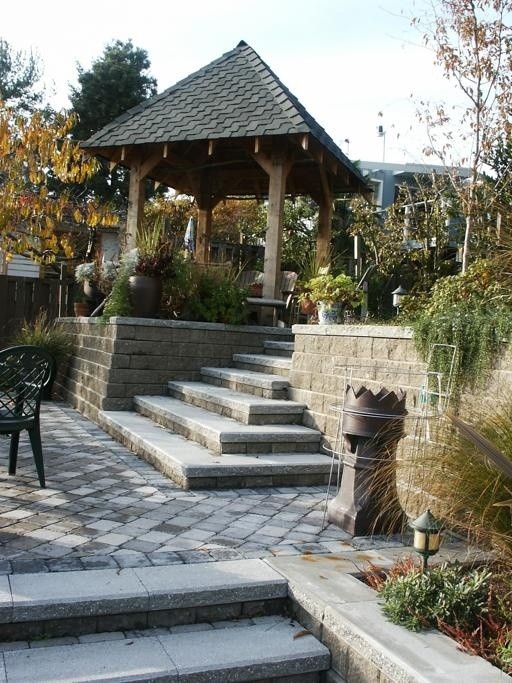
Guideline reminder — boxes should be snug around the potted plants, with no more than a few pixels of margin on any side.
[292,243,368,325]
[126,213,169,317]
[73,297,94,317]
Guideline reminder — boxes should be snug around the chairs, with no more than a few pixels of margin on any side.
[1,346,55,487]
[239,268,297,327]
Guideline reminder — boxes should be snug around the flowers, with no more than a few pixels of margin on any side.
[74,262,95,282]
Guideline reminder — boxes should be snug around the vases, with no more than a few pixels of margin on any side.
[83,280,94,296]
